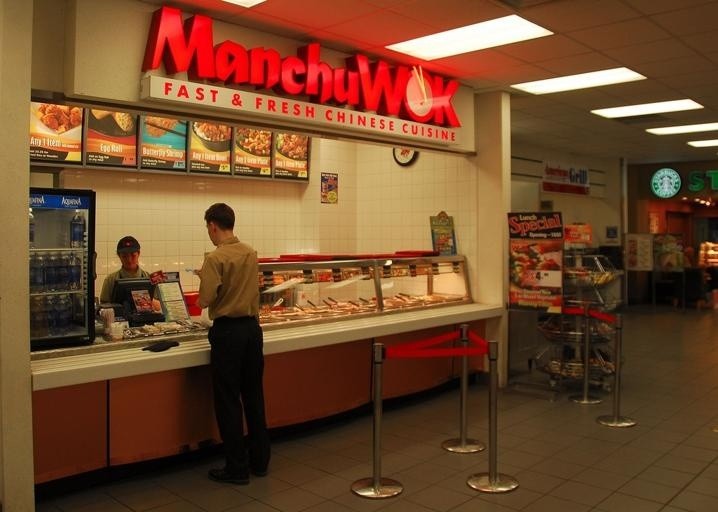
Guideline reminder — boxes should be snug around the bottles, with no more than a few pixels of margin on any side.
[29,208,35,249]
[42,253,55,291]
[28,252,43,293]
[33,294,73,337]
[68,251,80,290]
[70,209,85,248]
[56,252,67,291]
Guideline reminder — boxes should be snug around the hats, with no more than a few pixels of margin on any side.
[117,236,140,254]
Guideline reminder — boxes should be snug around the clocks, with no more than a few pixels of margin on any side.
[392,147,417,166]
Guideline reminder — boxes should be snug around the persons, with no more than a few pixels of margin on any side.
[195,203,270,485]
[99,235,161,311]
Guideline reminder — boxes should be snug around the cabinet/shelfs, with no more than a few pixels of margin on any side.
[530,253,622,392]
[27,254,504,484]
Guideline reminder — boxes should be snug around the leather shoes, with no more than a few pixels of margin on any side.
[247,463,269,477]
[208,467,249,485]
